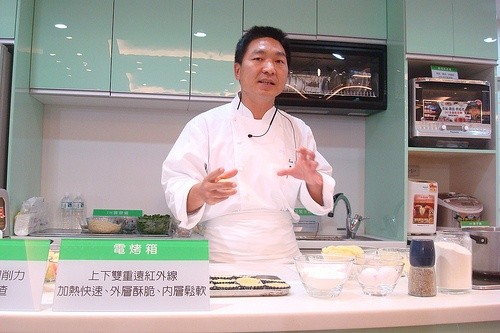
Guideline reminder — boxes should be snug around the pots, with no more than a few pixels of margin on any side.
[463,226,500,275]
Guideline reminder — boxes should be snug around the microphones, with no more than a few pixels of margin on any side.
[248,99,279,138]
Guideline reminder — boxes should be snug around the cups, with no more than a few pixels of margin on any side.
[409,238,436,297]
[435,230,473,294]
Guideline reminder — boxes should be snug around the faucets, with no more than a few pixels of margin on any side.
[327,192,370,239]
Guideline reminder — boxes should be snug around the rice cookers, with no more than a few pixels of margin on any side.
[438,192,484,231]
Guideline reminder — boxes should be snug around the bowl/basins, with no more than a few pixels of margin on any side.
[137,218,171,235]
[78,217,90,232]
[293,247,411,298]
[88,222,122,234]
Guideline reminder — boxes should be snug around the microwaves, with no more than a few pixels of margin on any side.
[274,34,387,117]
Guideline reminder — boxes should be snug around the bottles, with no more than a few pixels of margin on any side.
[61,192,85,230]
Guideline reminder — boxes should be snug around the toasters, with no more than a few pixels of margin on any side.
[409,177,438,235]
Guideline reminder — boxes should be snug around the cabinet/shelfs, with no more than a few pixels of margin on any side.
[0,0,500,235]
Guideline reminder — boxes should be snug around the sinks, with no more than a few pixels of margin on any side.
[296,234,345,242]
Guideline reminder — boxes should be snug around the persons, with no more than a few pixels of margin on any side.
[160,26,336,260]
